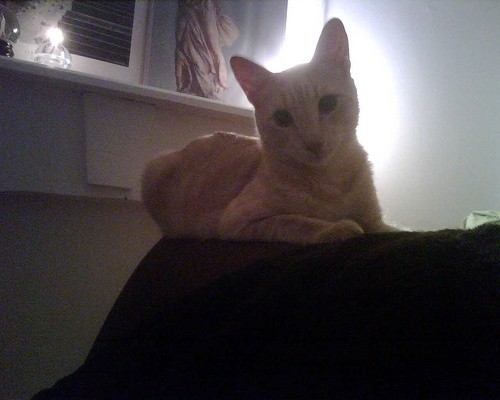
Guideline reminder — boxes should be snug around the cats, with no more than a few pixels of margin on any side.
[138,18,406,245]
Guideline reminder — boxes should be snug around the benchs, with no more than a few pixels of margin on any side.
[27,222,500,400]
